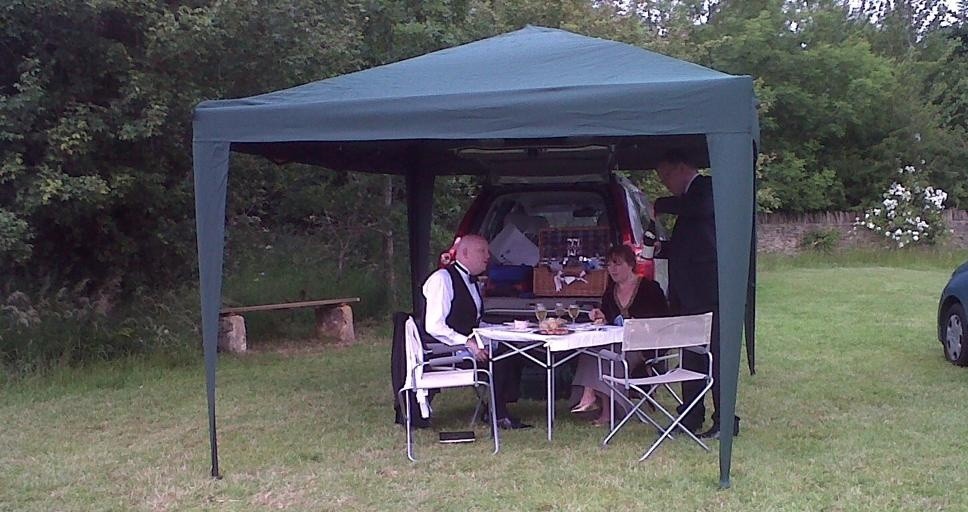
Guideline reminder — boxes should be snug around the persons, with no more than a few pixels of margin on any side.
[419,234,535,432]
[569,244,670,427]
[645,147,741,439]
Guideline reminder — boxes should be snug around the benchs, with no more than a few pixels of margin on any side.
[212,297,362,352]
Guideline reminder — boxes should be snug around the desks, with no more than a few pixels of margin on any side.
[471,323,627,444]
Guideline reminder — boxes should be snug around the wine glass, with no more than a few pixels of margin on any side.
[555,303,566,318]
[569,305,580,329]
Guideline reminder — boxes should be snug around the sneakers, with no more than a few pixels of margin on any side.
[588,419,611,428]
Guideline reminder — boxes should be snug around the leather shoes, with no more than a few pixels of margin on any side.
[694,419,739,440]
[659,417,703,436]
[481,407,533,431]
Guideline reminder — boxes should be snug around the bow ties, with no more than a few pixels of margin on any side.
[454,261,479,283]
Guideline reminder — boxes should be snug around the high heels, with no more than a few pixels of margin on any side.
[570,395,601,413]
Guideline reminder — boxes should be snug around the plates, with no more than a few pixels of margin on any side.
[508,328,533,332]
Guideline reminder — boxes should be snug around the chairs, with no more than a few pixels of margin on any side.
[397,313,501,464]
[596,311,714,463]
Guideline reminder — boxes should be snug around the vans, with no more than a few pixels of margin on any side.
[440,137,672,376]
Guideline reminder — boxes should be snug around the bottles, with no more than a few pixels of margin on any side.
[639,219,659,260]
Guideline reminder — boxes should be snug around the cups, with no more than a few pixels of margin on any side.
[535,304,548,320]
[514,319,529,329]
